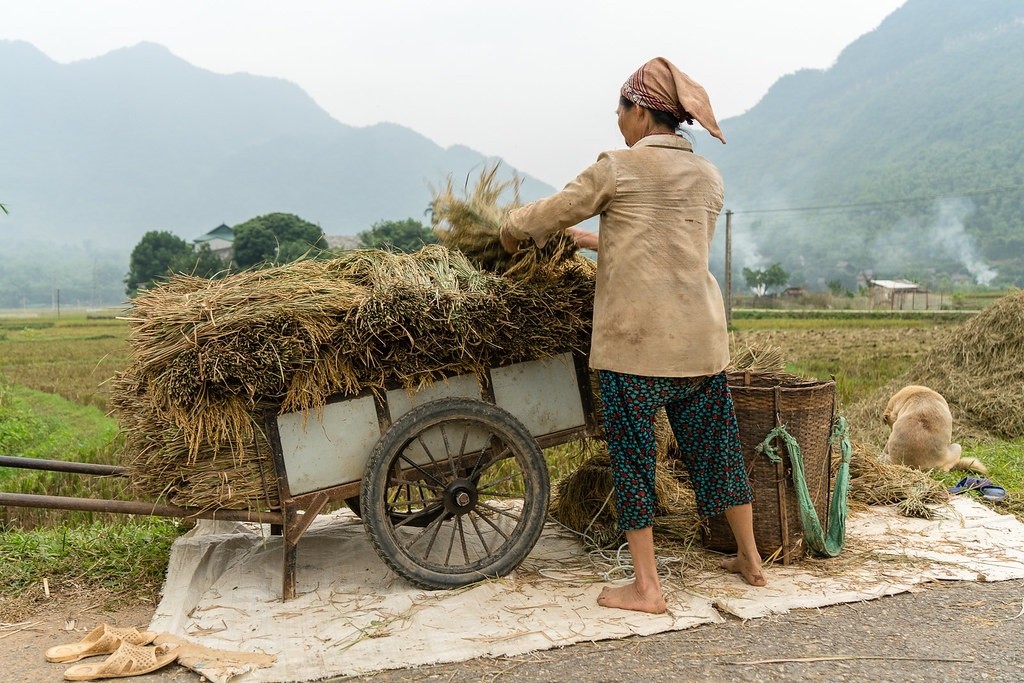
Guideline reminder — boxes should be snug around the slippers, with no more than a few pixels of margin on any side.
[980,485,1006,501]
[45,623,158,663]
[64,638,178,681]
[947,477,988,495]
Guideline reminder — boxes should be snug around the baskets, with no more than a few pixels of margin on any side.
[698,369,836,565]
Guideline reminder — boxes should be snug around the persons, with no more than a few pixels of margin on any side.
[499,57,768,614]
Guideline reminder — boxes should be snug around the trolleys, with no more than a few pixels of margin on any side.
[1,348,608,604]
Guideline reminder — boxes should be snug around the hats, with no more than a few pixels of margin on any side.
[621,57,726,144]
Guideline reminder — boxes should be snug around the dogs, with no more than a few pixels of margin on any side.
[883,385,991,476]
